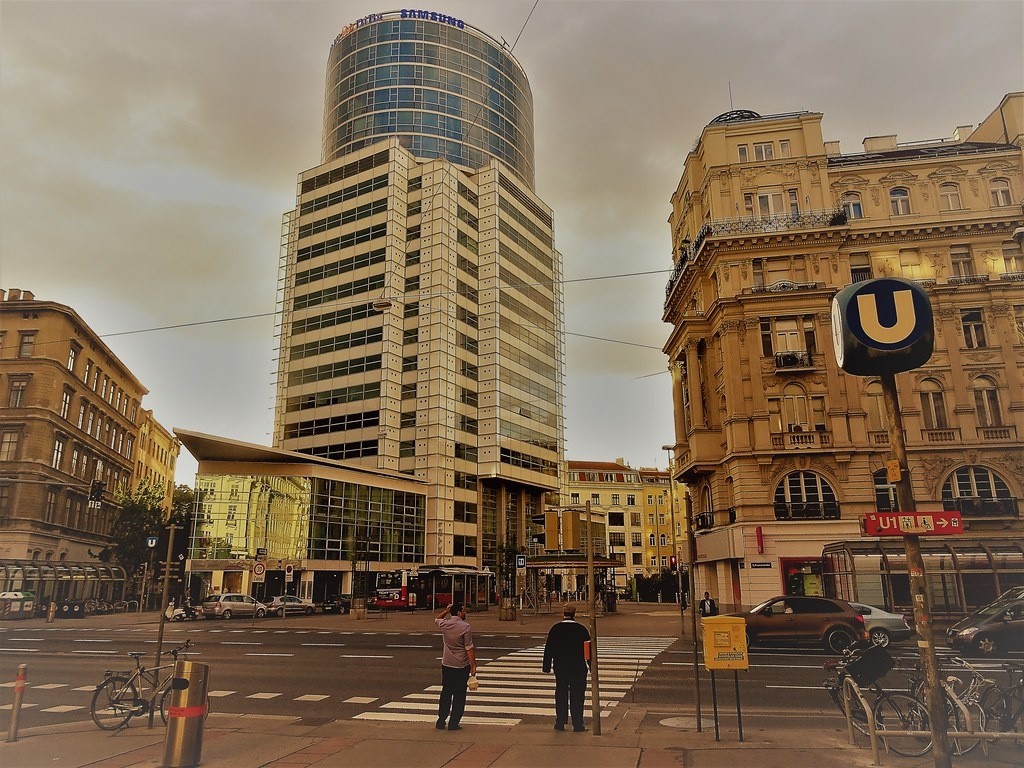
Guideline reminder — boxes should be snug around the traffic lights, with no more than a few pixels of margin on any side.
[88,480,105,503]
[669,555,676,570]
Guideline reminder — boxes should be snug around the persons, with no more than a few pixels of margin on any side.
[542,602,591,732]
[433,603,476,730]
[698,591,716,617]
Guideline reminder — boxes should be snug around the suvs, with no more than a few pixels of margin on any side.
[364,591,386,612]
[321,594,351,614]
[202,592,268,620]
[263,595,317,616]
[715,595,870,654]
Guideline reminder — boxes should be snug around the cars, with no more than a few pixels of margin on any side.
[846,601,912,648]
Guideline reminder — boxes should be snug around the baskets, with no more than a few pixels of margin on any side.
[843,643,896,689]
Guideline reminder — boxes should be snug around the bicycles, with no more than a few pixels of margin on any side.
[822,638,1024,758]
[90,637,211,731]
[74,597,115,616]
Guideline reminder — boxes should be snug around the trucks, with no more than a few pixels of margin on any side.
[997,587,1024,600]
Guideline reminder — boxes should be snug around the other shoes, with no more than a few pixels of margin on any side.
[554,723,564,731]
[436,725,445,729]
[448,725,462,730]
[574,726,585,732]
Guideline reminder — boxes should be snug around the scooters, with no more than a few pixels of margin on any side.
[165,597,200,621]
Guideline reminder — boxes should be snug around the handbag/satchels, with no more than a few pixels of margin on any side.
[467,676,479,691]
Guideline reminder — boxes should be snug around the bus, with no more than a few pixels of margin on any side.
[373,564,496,611]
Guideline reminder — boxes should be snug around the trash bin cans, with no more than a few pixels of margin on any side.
[0,592,24,620]
[20,592,36,619]
[163,660,210,767]
[46,601,56,622]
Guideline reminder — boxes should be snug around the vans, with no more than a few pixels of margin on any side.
[943,599,1024,658]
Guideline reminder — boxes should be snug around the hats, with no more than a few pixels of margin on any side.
[563,603,576,613]
